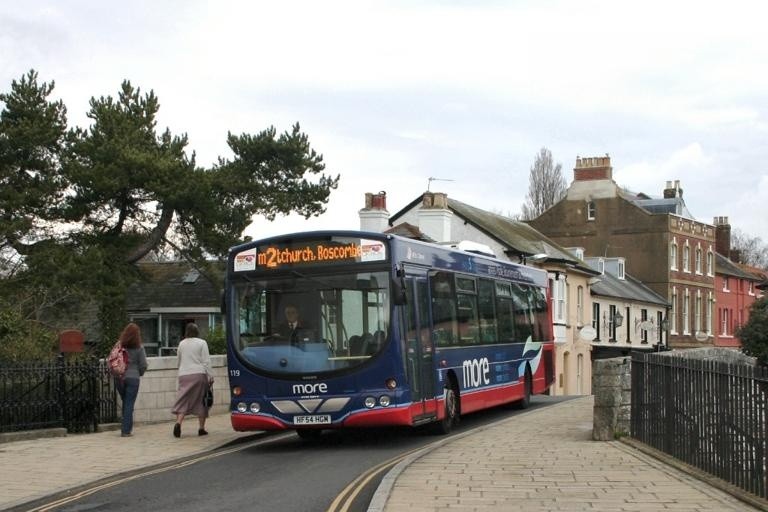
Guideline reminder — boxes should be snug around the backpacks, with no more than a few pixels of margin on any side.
[106,341,128,375]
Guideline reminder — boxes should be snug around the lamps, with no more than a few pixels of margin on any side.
[613,308,623,328]
[661,317,671,331]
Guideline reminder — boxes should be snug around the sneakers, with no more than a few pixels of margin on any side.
[121,430,134,437]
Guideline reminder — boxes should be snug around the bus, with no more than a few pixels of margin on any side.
[218,230,551,438]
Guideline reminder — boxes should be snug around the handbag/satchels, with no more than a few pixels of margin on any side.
[203,389,213,408]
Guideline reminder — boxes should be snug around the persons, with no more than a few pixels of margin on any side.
[171,323,214,437]
[110,323,148,437]
[284,304,313,332]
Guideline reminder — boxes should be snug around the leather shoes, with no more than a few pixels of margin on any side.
[173,423,181,437]
[199,429,208,435]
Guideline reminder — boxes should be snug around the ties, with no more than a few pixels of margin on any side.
[290,323,293,331]
